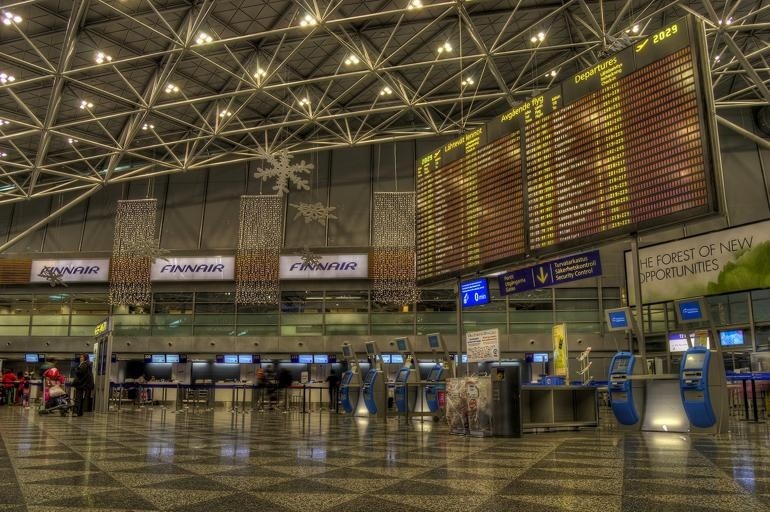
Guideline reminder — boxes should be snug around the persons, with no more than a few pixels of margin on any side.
[71,353,93,416]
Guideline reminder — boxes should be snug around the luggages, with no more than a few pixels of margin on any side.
[45,393,70,408]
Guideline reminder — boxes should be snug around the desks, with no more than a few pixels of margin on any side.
[520,384,598,432]
[406,382,445,423]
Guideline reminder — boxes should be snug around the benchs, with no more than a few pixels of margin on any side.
[108,398,135,412]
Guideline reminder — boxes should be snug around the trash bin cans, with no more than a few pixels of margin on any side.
[445,362,532,438]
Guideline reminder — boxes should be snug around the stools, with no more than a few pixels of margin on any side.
[728,385,742,416]
[597,387,612,434]
[292,394,300,412]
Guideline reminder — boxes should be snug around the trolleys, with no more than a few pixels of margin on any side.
[36,375,74,416]
